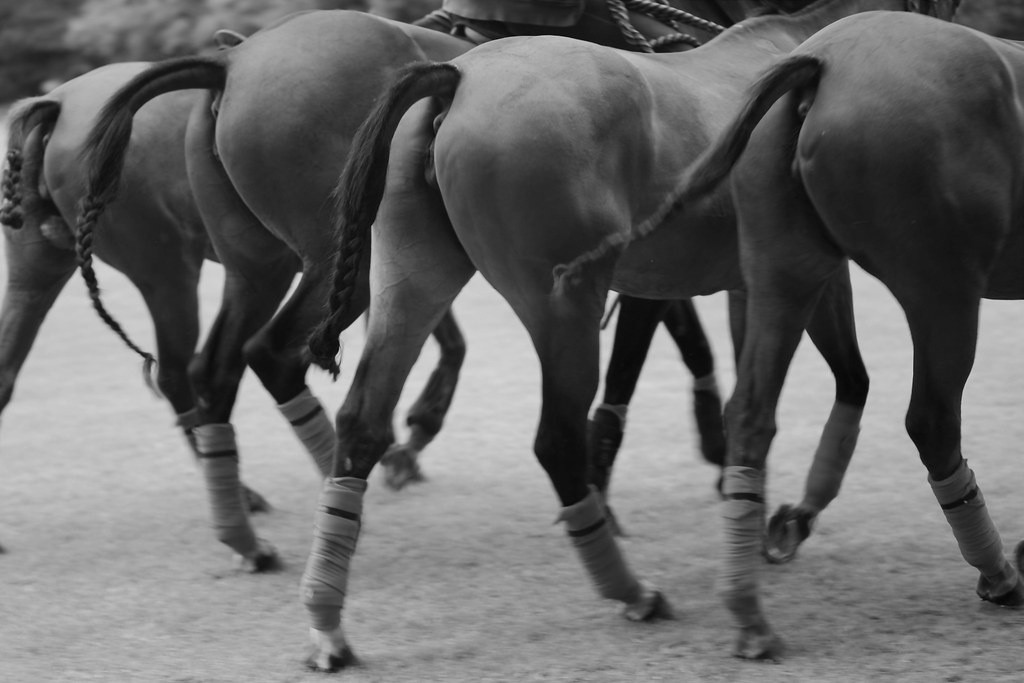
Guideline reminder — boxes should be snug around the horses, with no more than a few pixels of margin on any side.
[0,8,1023,673]
